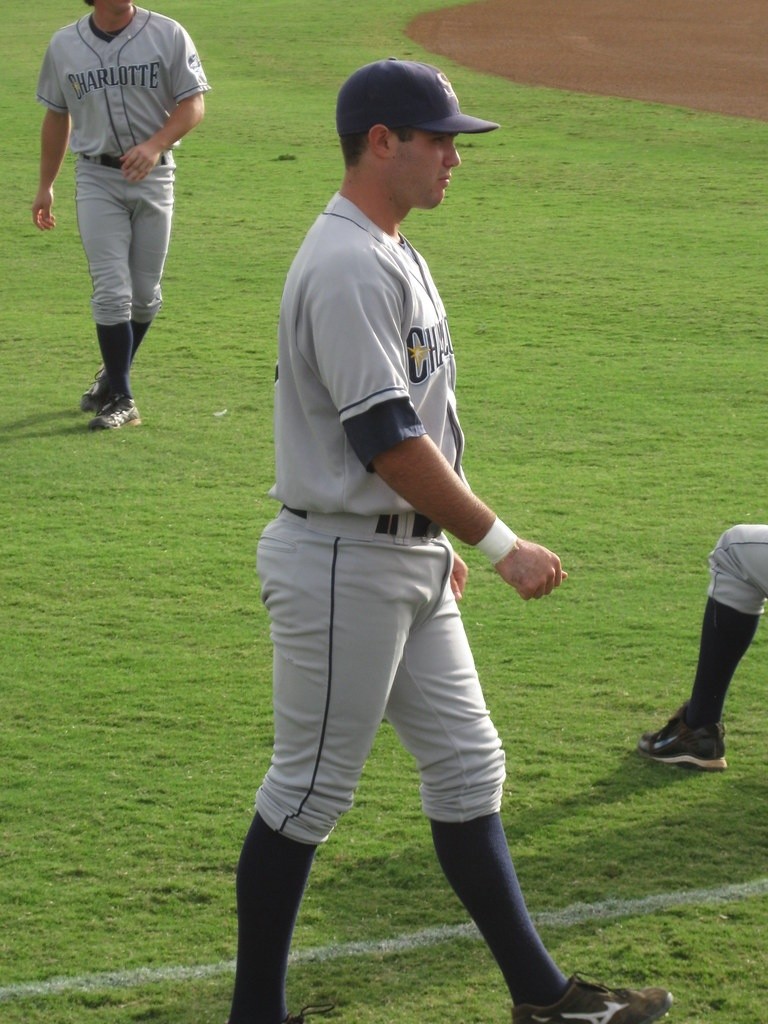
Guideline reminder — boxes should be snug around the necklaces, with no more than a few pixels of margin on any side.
[93,12,133,39]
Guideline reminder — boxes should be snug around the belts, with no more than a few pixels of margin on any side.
[82,154,165,169]
[283,503,442,539]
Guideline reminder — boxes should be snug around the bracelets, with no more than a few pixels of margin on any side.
[472,515,517,564]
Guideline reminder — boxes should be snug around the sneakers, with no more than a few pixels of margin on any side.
[511,971,673,1024]
[226,1004,334,1024]
[638,703,728,769]
[80,364,113,411]
[89,390,141,430]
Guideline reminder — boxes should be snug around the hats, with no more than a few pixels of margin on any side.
[336,57,500,136]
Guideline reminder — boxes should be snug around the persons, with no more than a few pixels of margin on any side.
[225,55,677,1024]
[637,523,767,772]
[33,0,214,434]
